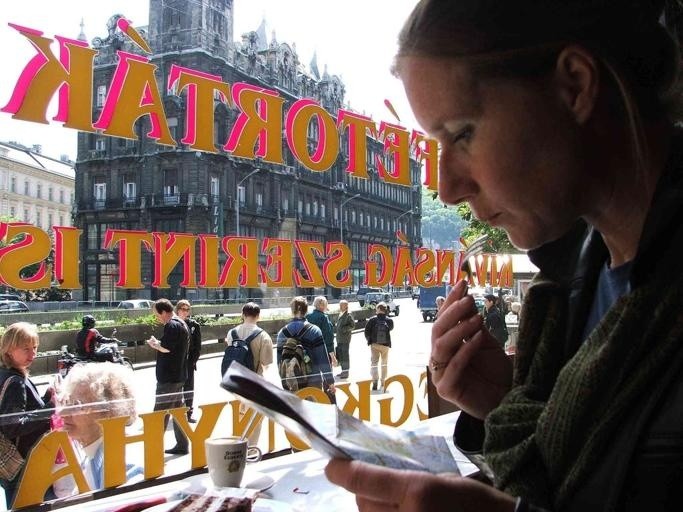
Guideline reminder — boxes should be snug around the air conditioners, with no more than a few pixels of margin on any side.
[335,181,344,190]
[286,166,294,175]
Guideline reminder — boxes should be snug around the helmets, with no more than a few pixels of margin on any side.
[82,315,95,327]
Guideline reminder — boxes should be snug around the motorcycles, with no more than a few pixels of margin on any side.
[57,327,134,378]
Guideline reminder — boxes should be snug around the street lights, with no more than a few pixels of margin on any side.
[235,169,260,300]
[395,210,412,264]
[338,194,360,298]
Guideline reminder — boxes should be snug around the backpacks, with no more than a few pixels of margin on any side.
[371,317,391,345]
[221,328,263,379]
[278,323,313,393]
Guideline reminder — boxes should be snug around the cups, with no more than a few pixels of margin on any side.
[204,437,261,489]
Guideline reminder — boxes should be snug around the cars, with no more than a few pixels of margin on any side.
[471,284,511,308]
[410,286,421,300]
[117,298,157,309]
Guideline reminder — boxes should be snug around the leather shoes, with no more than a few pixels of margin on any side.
[165,448,188,454]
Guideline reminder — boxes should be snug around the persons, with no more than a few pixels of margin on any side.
[482,293,509,351]
[324,0,681,512]
[144,298,201,454]
[276,294,355,407]
[364,301,394,392]
[1,313,145,512]
[226,302,274,455]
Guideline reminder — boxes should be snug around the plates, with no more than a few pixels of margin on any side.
[179,473,275,497]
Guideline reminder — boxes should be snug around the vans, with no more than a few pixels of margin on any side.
[0,294,23,301]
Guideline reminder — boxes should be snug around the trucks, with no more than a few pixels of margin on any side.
[418,281,454,321]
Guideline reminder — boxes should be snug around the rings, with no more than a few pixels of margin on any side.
[430,357,448,371]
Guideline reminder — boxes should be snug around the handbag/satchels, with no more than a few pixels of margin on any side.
[0,432,26,482]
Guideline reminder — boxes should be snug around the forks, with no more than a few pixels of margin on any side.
[456,232,490,302]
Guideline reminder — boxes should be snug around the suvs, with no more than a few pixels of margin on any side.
[356,287,388,306]
[364,292,399,316]
[0,299,29,312]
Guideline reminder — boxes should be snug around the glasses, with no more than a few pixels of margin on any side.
[179,307,189,312]
